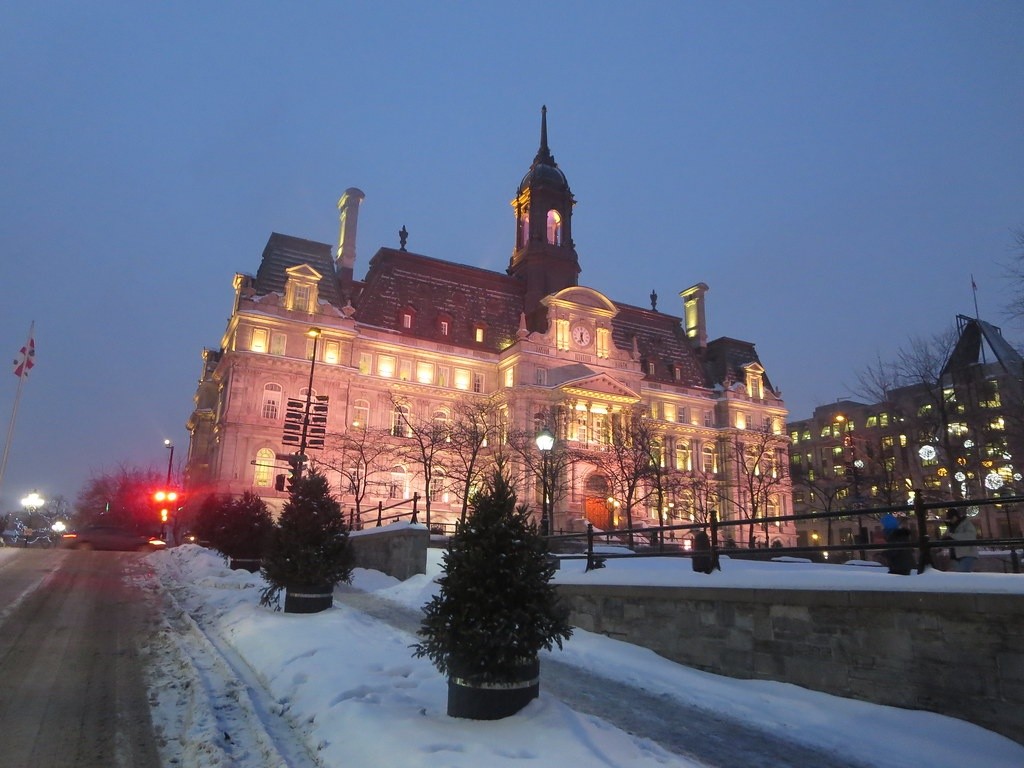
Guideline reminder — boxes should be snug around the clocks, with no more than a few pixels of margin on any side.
[572,326,590,347]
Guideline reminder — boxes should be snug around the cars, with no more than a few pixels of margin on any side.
[62,525,167,552]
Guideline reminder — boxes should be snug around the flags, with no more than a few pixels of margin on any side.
[11,329,36,378]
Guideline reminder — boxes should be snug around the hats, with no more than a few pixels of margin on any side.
[946,509,957,519]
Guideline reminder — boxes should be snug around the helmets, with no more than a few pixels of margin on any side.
[880,515,899,529]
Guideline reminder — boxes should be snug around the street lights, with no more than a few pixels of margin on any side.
[20,488,45,548]
[535,424,554,554]
[159,439,175,537]
[51,522,66,550]
[296,327,322,479]
[836,414,863,543]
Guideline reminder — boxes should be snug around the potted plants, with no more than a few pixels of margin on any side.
[260,455,354,614]
[214,491,275,573]
[406,450,577,720]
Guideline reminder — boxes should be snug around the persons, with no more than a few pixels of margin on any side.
[941,509,979,572]
